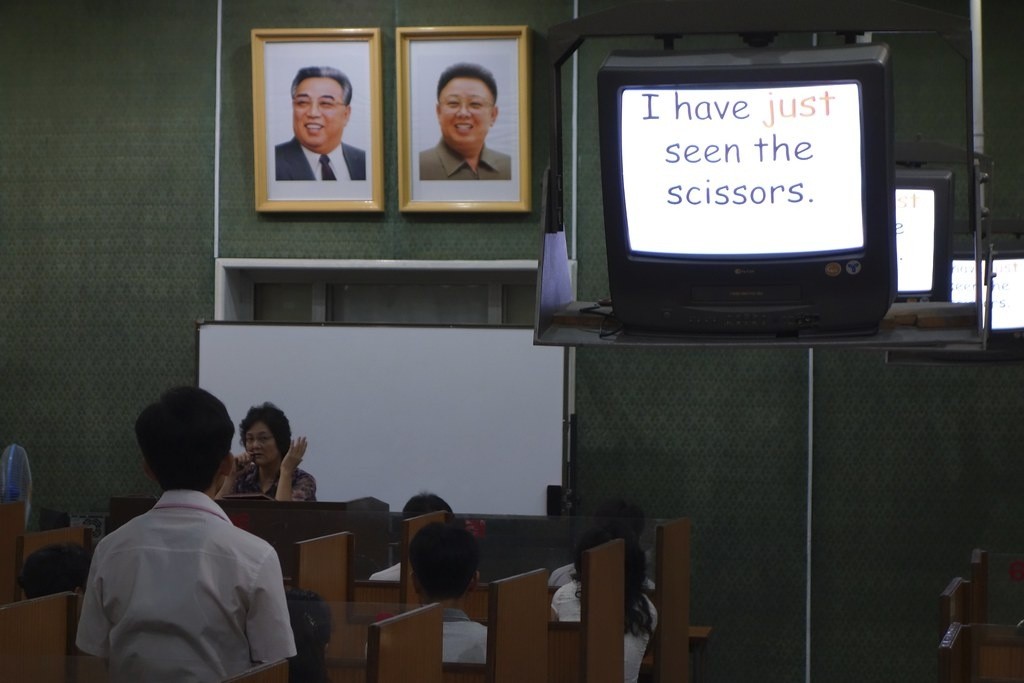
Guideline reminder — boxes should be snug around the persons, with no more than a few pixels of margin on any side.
[275,65,366,181]
[418,61,512,181]
[0,384,658,683]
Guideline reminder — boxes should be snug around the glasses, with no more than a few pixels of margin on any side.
[245,435,273,443]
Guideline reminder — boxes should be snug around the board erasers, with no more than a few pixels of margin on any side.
[546,485,562,516]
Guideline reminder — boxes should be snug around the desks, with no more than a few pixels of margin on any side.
[689,623,713,682]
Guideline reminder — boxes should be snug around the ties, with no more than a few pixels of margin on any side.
[319,153,338,182]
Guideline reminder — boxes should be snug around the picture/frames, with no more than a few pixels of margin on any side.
[395,25,532,213]
[250,27,384,213]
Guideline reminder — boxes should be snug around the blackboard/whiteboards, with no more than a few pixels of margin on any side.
[195,319,569,523]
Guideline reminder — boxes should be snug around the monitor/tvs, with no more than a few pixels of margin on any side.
[597,42,1023,340]
